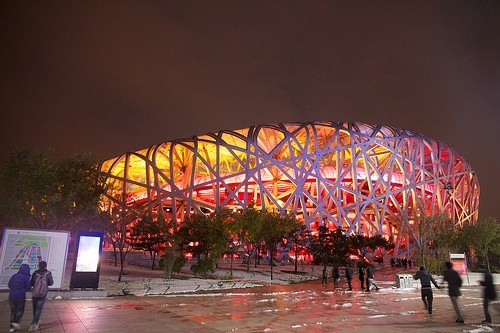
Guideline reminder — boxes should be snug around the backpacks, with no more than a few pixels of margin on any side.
[32,270,51,297]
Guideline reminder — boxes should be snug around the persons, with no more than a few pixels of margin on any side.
[477,265,494,323]
[28,260,54,331]
[442,262,464,323]
[322,254,412,292]
[412,266,440,314]
[8,264,32,332]
